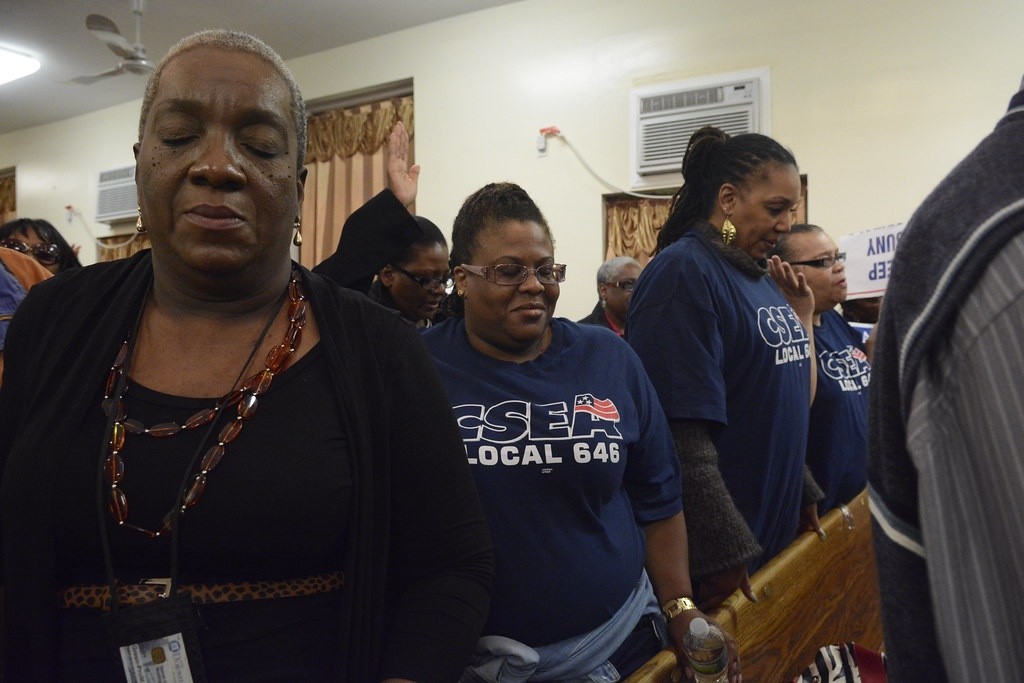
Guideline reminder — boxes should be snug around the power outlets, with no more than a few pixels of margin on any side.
[534,132,551,156]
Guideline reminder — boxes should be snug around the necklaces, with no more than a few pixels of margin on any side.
[103,267,307,537]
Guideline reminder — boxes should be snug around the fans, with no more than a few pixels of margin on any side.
[69,0,158,85]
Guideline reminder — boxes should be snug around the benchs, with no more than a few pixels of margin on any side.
[621,486,886,683]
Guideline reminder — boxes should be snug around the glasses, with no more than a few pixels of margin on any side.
[395,265,454,291]
[461,263,567,285]
[789,253,846,268]
[0,239,62,265]
[604,280,635,292]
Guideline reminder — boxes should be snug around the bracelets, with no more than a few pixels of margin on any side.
[661,597,698,623]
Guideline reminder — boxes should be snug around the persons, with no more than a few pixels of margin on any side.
[869,76,1024,683]
[625,124,827,612]
[423,182,744,683]
[760,222,880,517]
[575,256,643,342]
[0,30,497,683]
[0,218,85,380]
[310,121,452,334]
